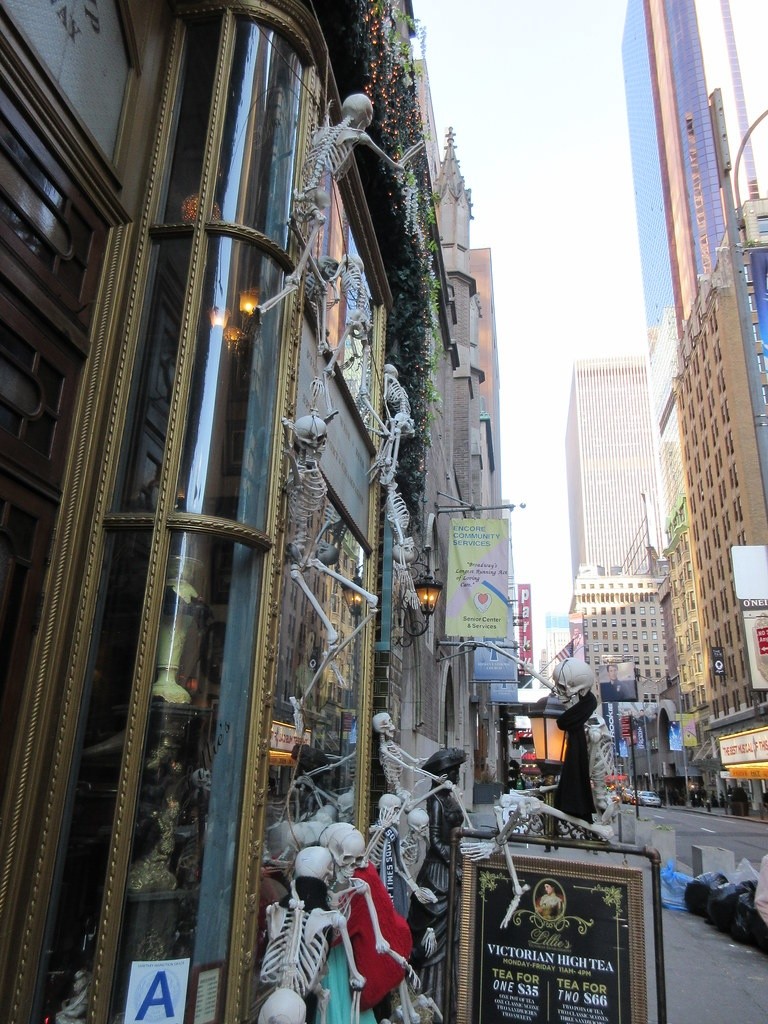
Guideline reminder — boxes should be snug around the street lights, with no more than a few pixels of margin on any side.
[676,676,697,809]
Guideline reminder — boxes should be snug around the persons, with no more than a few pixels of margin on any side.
[639,710,650,726]
[600,665,636,701]
[539,880,562,914]
[657,785,736,810]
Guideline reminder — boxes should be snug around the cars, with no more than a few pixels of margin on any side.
[620,788,661,807]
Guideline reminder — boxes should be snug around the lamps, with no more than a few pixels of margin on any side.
[391,569,443,649]
[210,285,261,358]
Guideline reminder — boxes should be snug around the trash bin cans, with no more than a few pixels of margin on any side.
[734,802,749,816]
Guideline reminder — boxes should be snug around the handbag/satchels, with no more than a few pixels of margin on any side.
[695,794,697,798]
